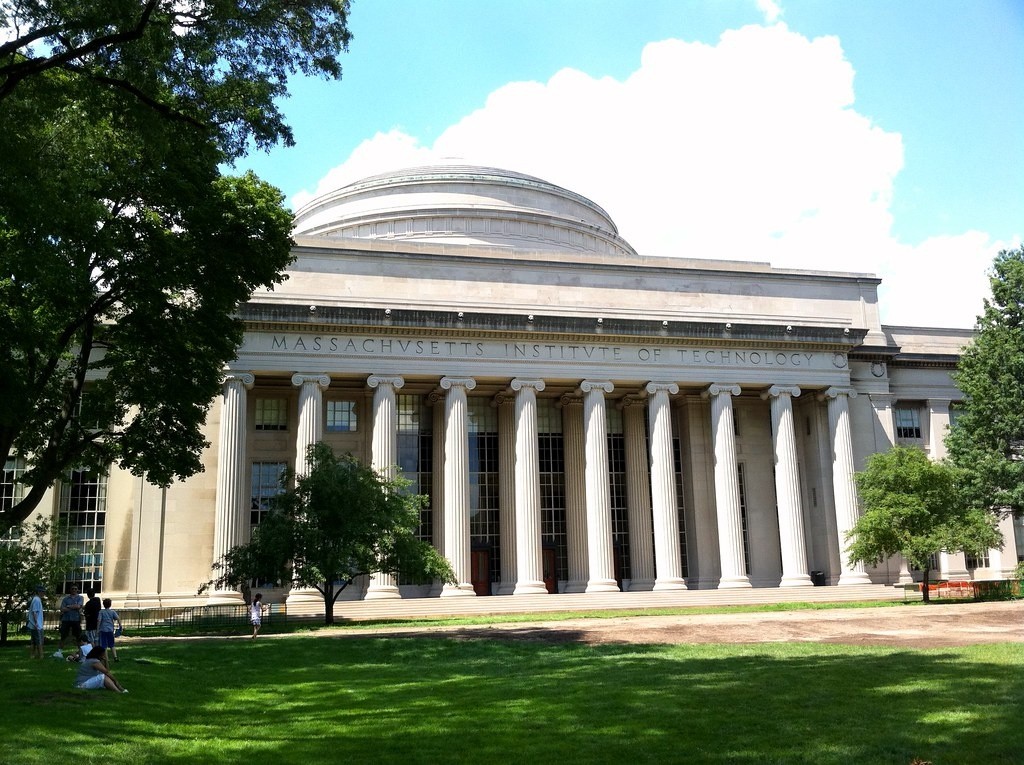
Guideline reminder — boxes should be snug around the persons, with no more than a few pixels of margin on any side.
[74,646,130,694]
[97,598,122,662]
[65,634,93,662]
[84,588,101,647]
[249,593,268,639]
[27,587,47,658]
[50,583,85,658]
